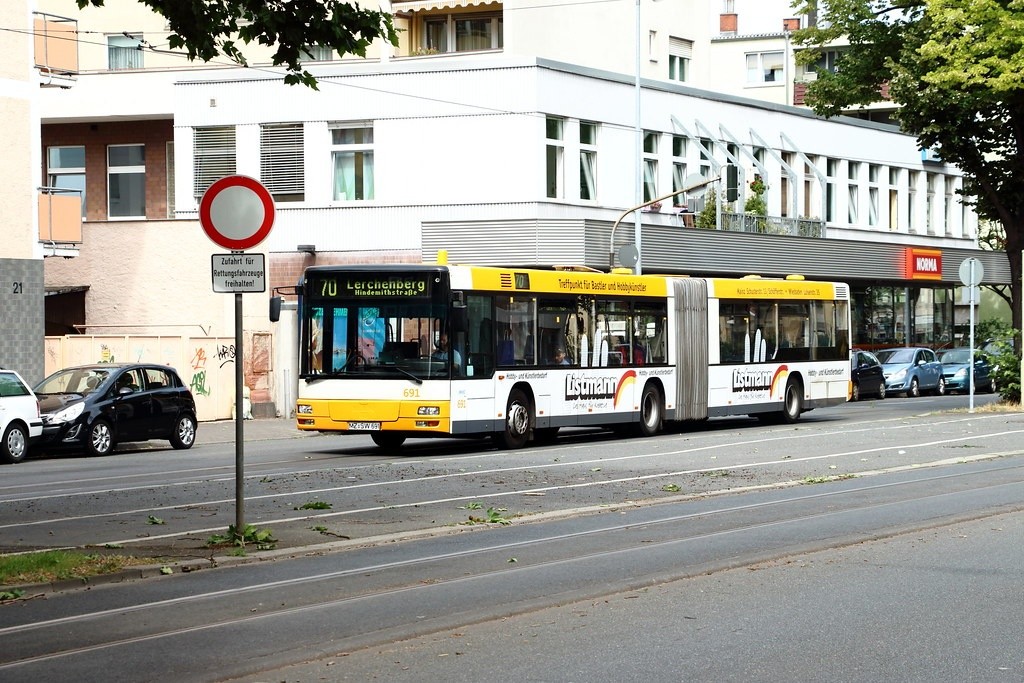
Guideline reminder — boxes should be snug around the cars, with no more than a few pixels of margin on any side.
[850,348,887,402]
[0,367,45,464]
[866,346,946,398]
[934,347,996,395]
[29,360,199,457]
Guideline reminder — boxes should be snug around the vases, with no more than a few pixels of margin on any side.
[651,206,660,212]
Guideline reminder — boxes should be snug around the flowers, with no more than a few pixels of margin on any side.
[652,201,665,207]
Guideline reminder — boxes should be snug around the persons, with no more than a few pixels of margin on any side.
[552,346,570,365]
[432,334,462,371]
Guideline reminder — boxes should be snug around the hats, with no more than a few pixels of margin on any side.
[551,347,565,354]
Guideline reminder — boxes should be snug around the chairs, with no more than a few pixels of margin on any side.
[117,373,139,392]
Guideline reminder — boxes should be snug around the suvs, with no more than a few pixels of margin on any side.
[981,337,1014,357]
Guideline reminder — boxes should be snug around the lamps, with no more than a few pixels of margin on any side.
[298,244,316,258]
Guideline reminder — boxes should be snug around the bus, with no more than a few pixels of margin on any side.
[267,247,853,451]
